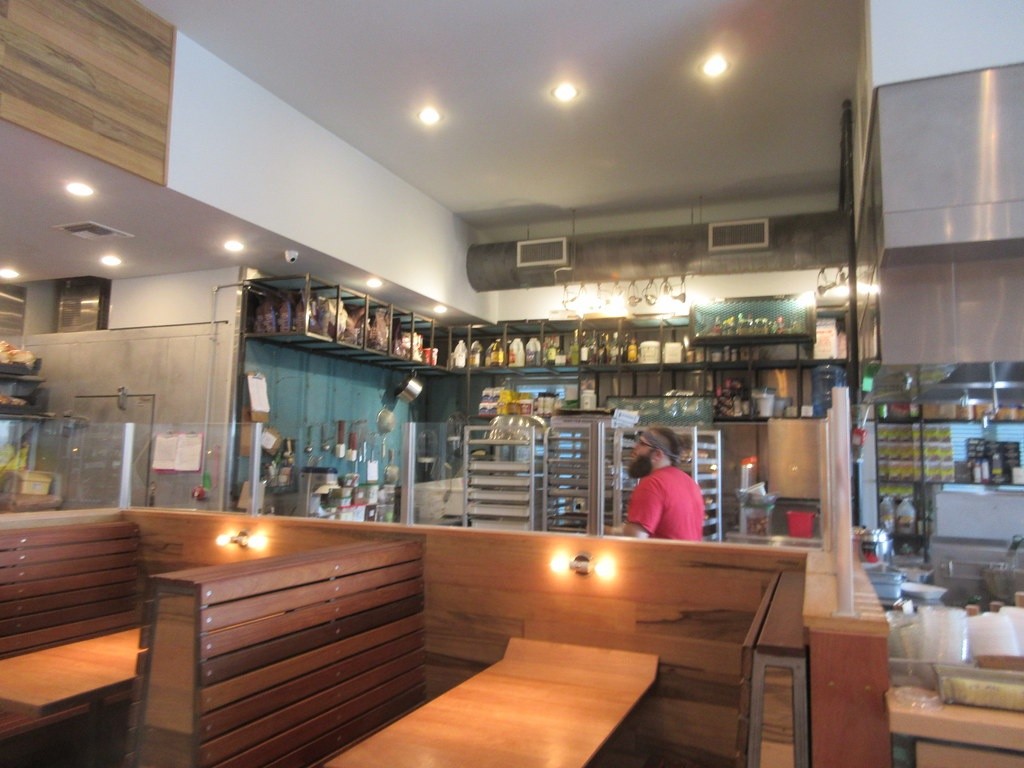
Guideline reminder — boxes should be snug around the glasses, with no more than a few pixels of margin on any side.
[637,437,656,450]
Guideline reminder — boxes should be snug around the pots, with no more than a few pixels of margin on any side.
[393,370,423,404]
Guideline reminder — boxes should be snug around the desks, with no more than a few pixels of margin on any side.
[0,624,151,768]
[324,637,659,768]
[884,686,1024,768]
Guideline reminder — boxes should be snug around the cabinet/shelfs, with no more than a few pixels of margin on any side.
[463,306,850,542]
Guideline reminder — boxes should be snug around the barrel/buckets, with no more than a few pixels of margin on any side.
[859,539,895,569]
[786,510,814,537]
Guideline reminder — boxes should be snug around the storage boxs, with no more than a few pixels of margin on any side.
[4,472,52,495]
[866,565,903,599]
[301,467,394,522]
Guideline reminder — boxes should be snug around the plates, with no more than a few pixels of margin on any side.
[901,581,946,599]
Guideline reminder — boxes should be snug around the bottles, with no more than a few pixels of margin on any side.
[721,313,768,336]
[569,327,639,363]
[453,338,480,366]
[879,495,914,535]
[484,337,559,367]
[813,360,846,419]
[972,458,988,484]
[966,591,1024,616]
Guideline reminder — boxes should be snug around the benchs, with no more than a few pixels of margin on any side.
[736,569,781,768]
[119,544,427,768]
[0,521,143,747]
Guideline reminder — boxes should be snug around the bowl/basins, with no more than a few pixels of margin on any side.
[740,482,765,497]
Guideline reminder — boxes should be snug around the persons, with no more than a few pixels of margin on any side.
[604,426,705,541]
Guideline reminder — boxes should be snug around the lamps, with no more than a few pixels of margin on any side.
[645,295,657,305]
[818,268,848,297]
[569,554,593,575]
[229,531,249,548]
[672,293,685,303]
[628,296,642,307]
